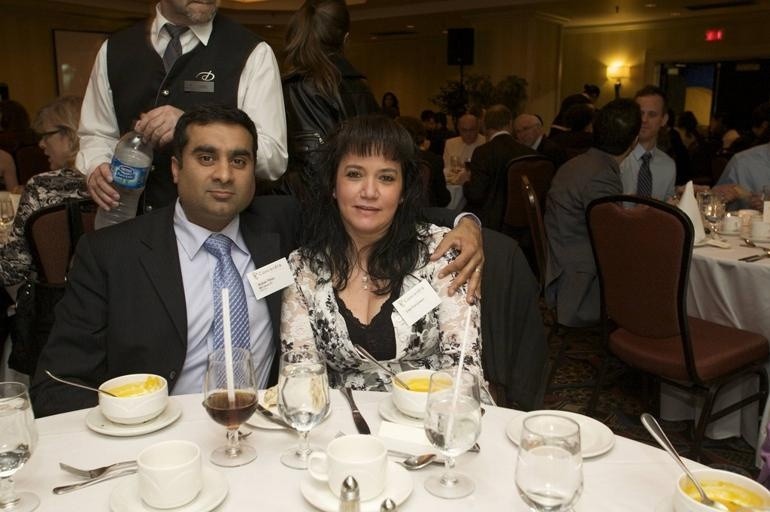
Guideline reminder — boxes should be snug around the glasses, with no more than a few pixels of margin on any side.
[516,121,541,136]
[36,128,66,143]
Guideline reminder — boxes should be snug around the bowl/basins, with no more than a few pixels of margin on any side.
[96,373,168,424]
[391,369,454,419]
[674,467,769,508]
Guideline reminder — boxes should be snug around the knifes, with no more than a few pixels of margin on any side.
[339,388,374,435]
[235,386,297,429]
[52,473,136,499]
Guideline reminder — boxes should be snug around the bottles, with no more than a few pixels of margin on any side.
[94,131,154,234]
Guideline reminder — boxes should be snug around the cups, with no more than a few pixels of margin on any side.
[137,440,203,507]
[739,210,759,226]
[752,222,770,239]
[722,216,742,235]
[277,350,329,470]
[327,433,389,502]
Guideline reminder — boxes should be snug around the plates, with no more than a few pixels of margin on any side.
[239,389,331,429]
[507,409,616,458]
[298,463,416,512]
[110,465,231,511]
[84,402,184,437]
[243,388,292,432]
[378,391,486,427]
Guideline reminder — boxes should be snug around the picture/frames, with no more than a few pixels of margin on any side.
[53,29,109,97]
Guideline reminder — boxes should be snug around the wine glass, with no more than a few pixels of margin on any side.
[1,381,41,511]
[426,370,482,500]
[515,415,581,512]
[203,346,259,468]
[701,192,728,244]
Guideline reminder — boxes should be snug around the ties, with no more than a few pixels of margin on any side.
[203,234,250,388]
[638,152,653,201]
[161,24,191,73]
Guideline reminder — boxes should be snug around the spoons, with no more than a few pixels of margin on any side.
[352,343,413,396]
[742,238,770,252]
[639,412,727,509]
[398,453,437,471]
[337,475,360,510]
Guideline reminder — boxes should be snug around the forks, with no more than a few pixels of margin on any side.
[59,458,138,479]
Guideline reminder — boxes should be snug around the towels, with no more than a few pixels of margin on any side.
[675,179,706,243]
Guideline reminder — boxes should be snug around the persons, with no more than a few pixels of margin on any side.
[278,115,497,406]
[75,0,289,216]
[282,0,379,196]
[653,99,770,150]
[543,97,642,330]
[621,84,676,208]
[395,115,451,206]
[0,93,89,370]
[515,114,555,160]
[420,109,460,145]
[444,115,486,182]
[29,105,485,419]
[711,144,770,212]
[383,93,399,116]
[456,106,541,220]
[551,83,599,145]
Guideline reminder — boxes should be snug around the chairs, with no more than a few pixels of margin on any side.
[0,198,99,377]
[586,194,770,458]
[481,227,546,411]
[503,154,553,233]
[242,195,304,236]
[519,174,629,393]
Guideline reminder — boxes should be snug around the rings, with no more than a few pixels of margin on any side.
[474,269,482,274]
